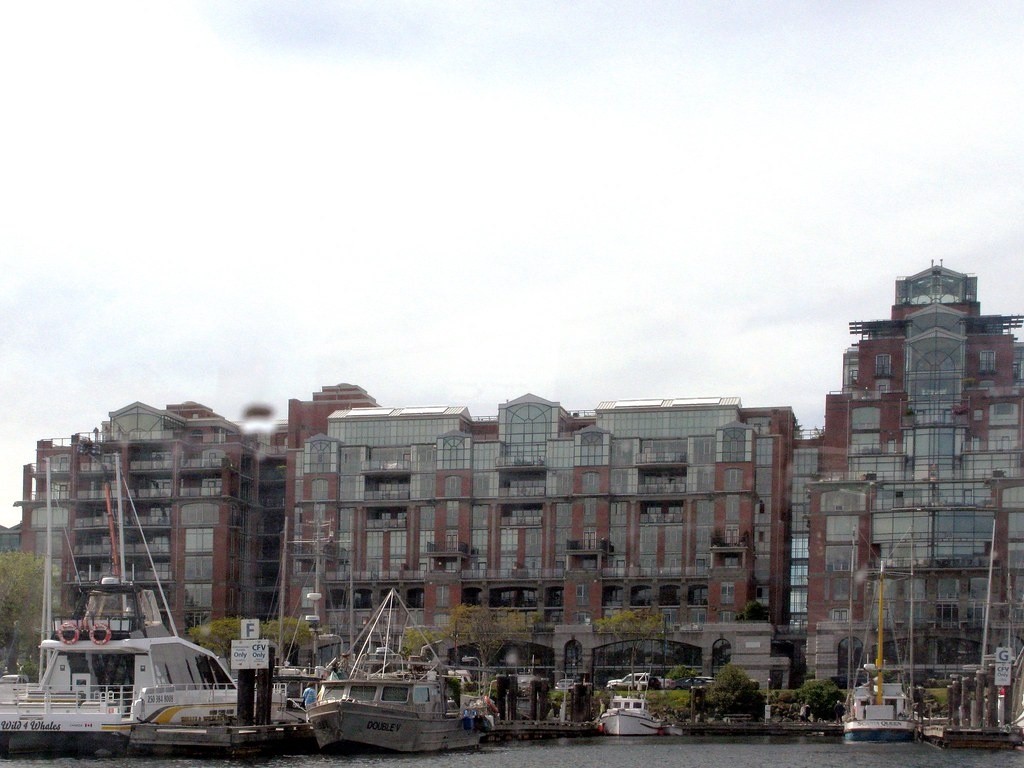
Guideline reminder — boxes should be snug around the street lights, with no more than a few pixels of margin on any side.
[421,639,444,657]
[319,633,343,660]
[552,670,567,727]
[462,656,481,696]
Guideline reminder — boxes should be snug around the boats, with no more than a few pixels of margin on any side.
[0,450,288,755]
[304,584,486,750]
[600,695,663,736]
[841,525,924,743]
[664,724,684,737]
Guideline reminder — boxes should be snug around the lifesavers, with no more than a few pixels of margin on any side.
[89,623,111,645]
[58,622,79,645]
[480,714,487,721]
[485,696,498,713]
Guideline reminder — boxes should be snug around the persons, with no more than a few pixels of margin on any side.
[599,698,606,714]
[834,700,844,725]
[303,682,317,723]
[800,702,811,723]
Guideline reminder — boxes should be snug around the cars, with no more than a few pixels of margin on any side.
[555,672,716,692]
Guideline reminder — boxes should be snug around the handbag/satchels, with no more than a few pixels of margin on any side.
[301,700,305,708]
